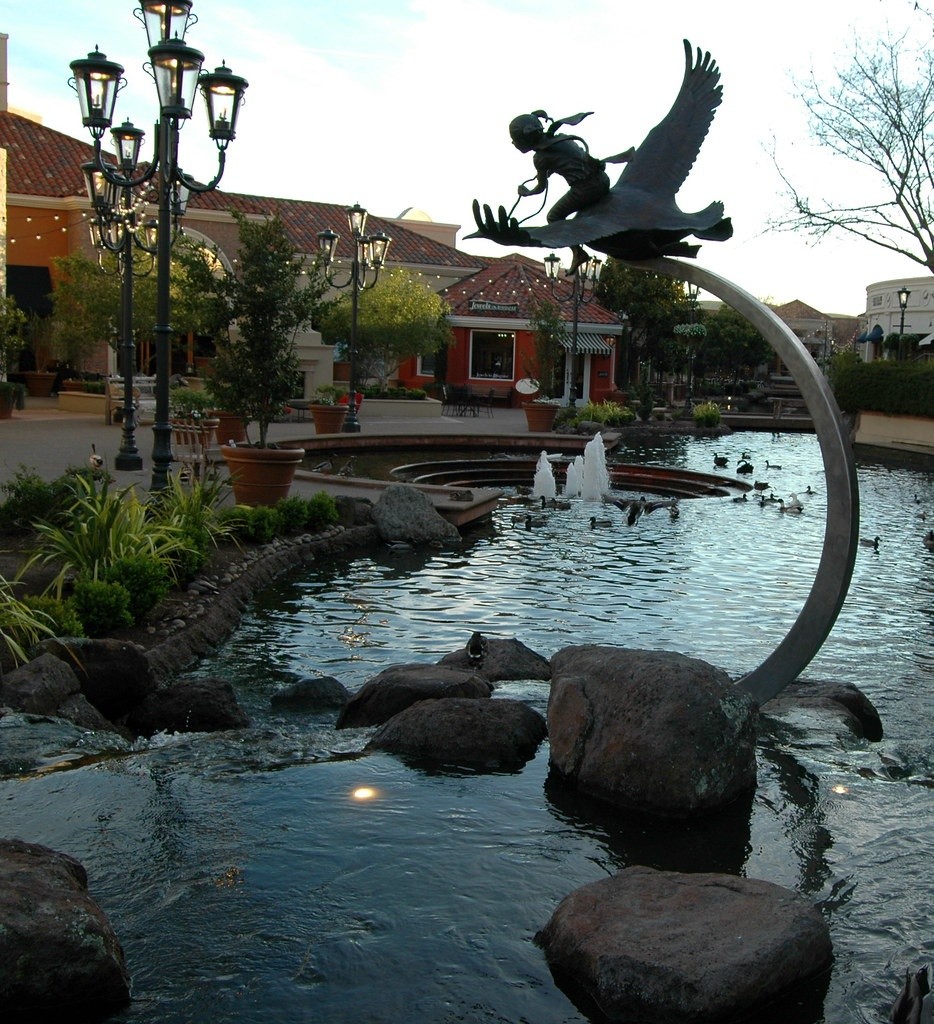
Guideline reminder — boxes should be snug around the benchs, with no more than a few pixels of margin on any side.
[768,397,807,419]
[105,376,158,426]
[471,386,513,408]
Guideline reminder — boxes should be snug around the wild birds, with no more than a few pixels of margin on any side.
[460,36,733,261]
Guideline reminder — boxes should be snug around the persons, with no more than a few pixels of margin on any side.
[509,111,635,277]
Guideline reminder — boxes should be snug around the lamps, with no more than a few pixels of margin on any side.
[602,333,615,359]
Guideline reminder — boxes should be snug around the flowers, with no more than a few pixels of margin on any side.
[521,294,564,404]
[311,385,345,406]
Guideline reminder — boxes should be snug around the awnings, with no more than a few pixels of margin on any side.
[855,331,867,343]
[551,332,613,356]
[865,328,881,342]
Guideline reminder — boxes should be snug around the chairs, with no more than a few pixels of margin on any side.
[441,383,495,418]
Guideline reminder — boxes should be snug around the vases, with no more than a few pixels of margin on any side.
[523,403,560,431]
[308,405,348,434]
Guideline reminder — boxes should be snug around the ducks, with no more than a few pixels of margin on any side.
[732,478,816,515]
[736,452,782,474]
[535,495,571,510]
[586,490,680,530]
[859,535,883,547]
[911,493,922,504]
[915,510,928,521]
[922,529,934,549]
[709,451,729,468]
[510,513,548,528]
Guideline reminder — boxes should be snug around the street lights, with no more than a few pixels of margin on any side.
[67,0,248,584]
[80,117,193,472]
[829,337,836,371]
[316,200,391,432]
[544,245,602,431]
[897,285,911,360]
[678,281,700,416]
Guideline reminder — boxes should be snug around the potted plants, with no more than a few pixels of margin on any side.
[163,204,353,509]
[24,306,85,397]
[333,342,351,380]
[689,398,725,428]
[170,385,253,445]
[0,286,28,420]
